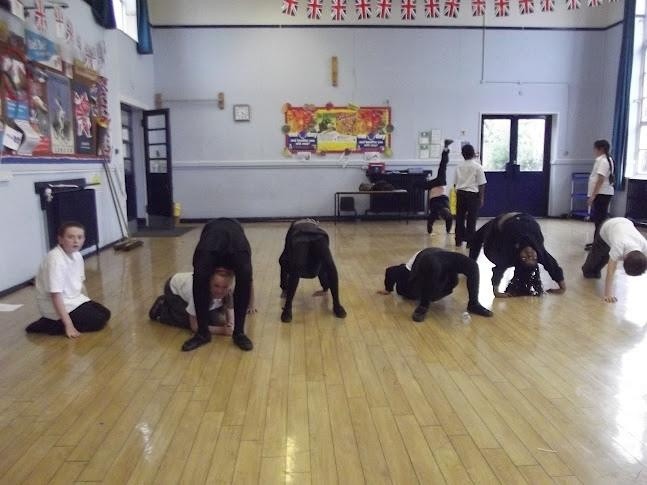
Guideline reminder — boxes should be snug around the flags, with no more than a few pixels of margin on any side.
[330,0,347,21]
[566,0,582,10]
[470,0,485,17]
[494,0,509,17]
[353,0,371,20]
[279,0,297,15]
[518,0,533,15]
[585,0,602,6]
[424,0,439,18]
[399,0,415,20]
[539,0,554,14]
[304,0,322,19]
[374,0,391,19]
[442,0,461,19]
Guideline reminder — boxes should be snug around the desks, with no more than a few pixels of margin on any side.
[333,191,409,225]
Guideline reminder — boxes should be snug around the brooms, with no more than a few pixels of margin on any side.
[99,144,145,252]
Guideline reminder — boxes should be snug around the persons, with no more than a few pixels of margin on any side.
[581,137,617,252]
[467,210,567,297]
[147,267,234,338]
[276,218,348,323]
[376,246,493,324]
[414,138,454,237]
[581,216,645,303]
[181,215,259,352]
[451,143,488,250]
[24,220,111,339]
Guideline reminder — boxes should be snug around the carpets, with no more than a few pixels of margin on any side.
[130,226,196,237]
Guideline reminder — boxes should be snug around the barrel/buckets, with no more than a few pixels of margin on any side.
[174,202,181,216]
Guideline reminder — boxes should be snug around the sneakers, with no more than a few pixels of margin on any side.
[282,307,292,322]
[583,265,602,278]
[232,330,253,350]
[413,304,428,321]
[26,317,50,333]
[468,302,493,318]
[149,296,167,320]
[183,331,211,351]
[444,140,453,146]
[333,304,347,318]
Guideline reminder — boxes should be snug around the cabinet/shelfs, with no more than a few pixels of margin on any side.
[570,172,592,217]
